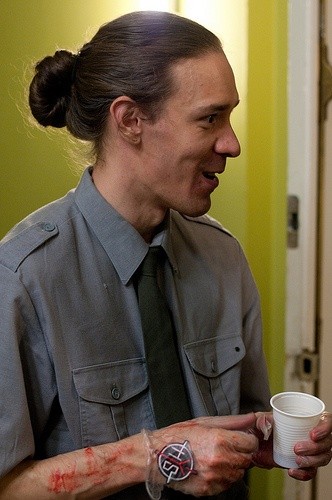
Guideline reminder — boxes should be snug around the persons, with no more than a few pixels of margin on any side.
[1,10,331,500]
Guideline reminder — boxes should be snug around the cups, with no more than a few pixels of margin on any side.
[270,392,325,468]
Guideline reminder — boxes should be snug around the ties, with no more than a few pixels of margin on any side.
[138,248,193,431]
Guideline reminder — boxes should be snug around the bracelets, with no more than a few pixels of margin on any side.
[141,429,196,497]
